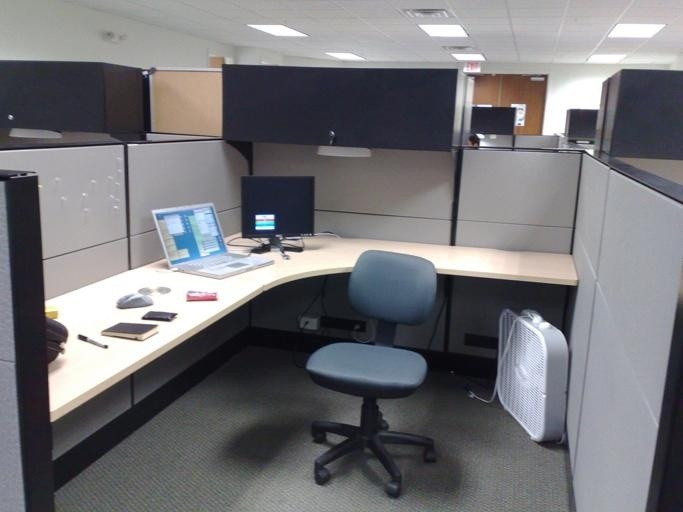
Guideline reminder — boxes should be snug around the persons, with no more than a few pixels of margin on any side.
[468,132,480,149]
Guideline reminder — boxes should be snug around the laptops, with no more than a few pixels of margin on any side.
[151,203,274,279]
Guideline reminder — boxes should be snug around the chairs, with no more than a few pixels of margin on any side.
[305,248,438,498]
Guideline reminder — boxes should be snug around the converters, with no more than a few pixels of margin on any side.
[299,313,321,331]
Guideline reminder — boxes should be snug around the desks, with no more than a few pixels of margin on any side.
[45,232,578,423]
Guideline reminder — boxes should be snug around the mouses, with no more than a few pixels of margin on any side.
[117,294,152,309]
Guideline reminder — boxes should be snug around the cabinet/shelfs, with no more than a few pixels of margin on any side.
[0,59,683,163]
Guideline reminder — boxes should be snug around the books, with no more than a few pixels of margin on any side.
[185,290,217,301]
[100,322,160,341]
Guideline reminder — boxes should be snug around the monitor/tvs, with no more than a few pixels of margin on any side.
[240,176,314,253]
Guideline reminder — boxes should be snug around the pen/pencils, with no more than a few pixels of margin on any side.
[78,335,108,348]
